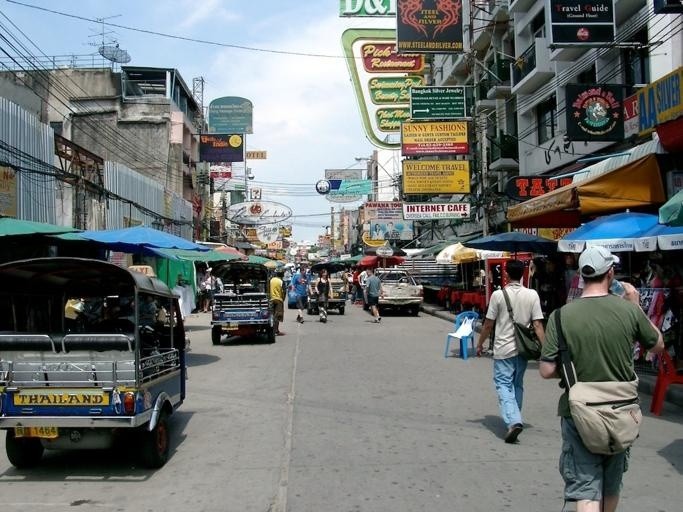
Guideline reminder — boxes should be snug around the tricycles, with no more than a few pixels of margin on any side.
[208,261,277,346]
[306,261,349,315]
[0,254,188,472]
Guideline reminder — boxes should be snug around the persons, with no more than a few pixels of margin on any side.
[201,271,217,312]
[270,269,383,336]
[475,259,546,443]
[384,222,401,239]
[371,224,384,241]
[540,246,665,512]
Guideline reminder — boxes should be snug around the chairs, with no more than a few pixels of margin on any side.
[650,349,683,416]
[445,311,480,359]
[214,292,267,307]
[0,330,140,385]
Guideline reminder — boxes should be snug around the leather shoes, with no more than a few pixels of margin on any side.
[277,332,285,336]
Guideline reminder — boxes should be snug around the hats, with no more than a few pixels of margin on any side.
[578,246,620,278]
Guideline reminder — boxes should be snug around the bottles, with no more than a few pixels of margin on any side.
[607,279,626,299]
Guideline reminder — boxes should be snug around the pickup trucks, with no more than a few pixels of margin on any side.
[363,268,424,315]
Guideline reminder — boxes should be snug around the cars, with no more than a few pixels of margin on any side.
[288,272,314,307]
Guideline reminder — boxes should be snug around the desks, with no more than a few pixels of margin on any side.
[438,287,486,313]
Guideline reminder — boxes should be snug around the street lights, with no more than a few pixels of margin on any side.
[356,157,402,200]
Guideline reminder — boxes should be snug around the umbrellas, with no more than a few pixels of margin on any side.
[84,223,249,269]
[435,209,683,296]
[0,217,82,241]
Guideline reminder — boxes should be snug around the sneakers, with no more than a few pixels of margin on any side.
[375,317,381,322]
[297,315,328,324]
[505,424,523,443]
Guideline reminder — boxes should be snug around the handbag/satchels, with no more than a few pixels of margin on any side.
[513,318,543,361]
[554,308,643,456]
